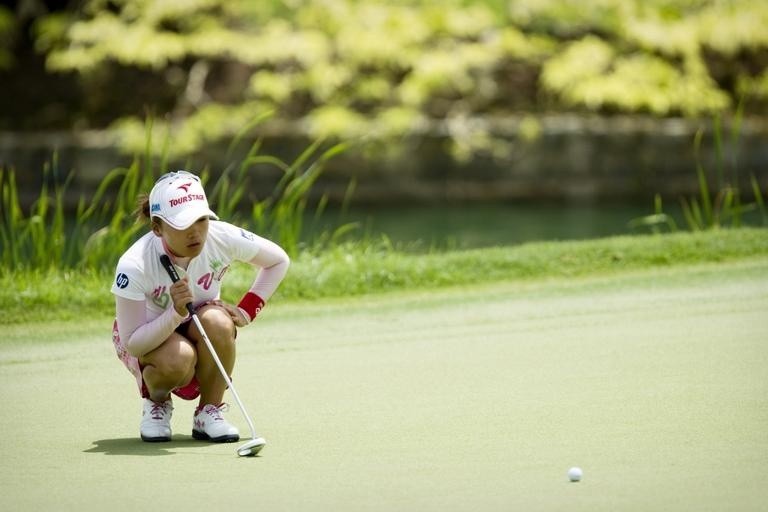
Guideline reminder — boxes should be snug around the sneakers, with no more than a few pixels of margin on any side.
[139,394,173,443]
[191,402,240,441]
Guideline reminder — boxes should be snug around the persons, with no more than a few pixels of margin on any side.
[111,168,291,444]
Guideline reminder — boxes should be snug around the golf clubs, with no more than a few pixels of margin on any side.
[159,254,265,456]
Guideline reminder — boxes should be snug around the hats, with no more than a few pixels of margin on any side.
[149,170,220,232]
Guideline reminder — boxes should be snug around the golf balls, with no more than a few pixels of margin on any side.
[569,468,580,481]
